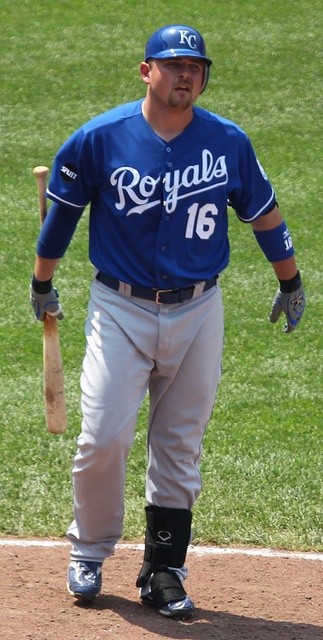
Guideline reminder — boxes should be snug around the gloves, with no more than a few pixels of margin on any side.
[267,270,305,335]
[30,274,65,323]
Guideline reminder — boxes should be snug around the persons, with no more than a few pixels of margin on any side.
[30,24,306,619]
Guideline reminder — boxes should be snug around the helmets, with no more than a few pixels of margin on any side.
[144,25,213,66]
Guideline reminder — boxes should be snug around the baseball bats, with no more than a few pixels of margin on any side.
[32,166,66,434]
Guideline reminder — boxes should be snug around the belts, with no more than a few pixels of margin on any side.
[94,269,218,308]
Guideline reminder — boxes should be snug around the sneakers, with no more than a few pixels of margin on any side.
[139,564,196,621]
[66,554,102,601]
